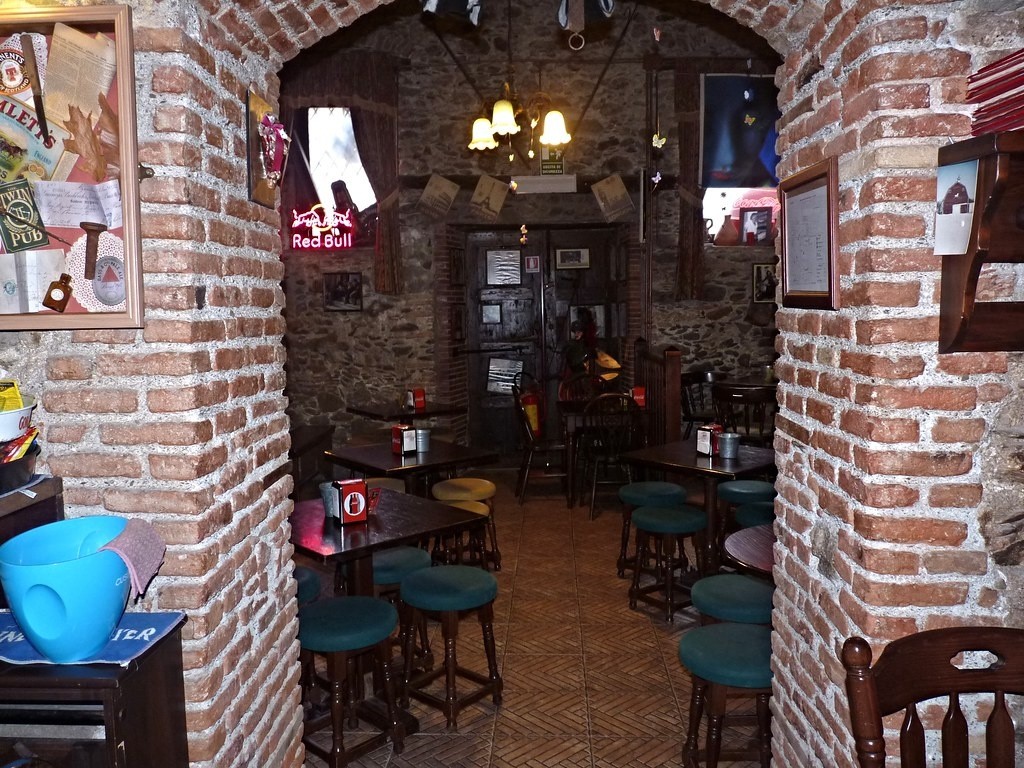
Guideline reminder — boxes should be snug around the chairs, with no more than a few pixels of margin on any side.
[513,371,727,521]
[842,626,1024,768]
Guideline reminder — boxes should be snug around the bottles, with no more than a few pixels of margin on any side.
[703,218,713,243]
[357,494,360,512]
[352,494,357,514]
[43,273,73,312]
[713,215,738,246]
[349,494,353,513]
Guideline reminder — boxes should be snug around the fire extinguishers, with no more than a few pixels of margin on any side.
[514,371,541,437]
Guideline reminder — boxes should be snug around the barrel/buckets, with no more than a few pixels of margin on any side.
[0,516,132,663]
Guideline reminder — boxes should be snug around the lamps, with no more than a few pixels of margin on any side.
[466,77,571,169]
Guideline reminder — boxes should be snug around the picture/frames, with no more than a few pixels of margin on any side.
[556,299,573,318]
[569,303,611,339]
[450,249,540,396]
[322,272,363,312]
[555,248,593,270]
[697,73,840,311]
[0,5,143,328]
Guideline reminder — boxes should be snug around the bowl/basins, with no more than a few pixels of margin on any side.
[0,443,41,496]
[0,399,39,442]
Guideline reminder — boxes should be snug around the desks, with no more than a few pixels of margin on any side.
[710,378,775,439]
[619,438,774,580]
[287,489,489,737]
[555,400,656,509]
[322,439,498,498]
[346,399,468,426]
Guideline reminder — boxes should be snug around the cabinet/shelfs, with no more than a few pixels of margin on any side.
[289,426,336,501]
[0,477,191,768]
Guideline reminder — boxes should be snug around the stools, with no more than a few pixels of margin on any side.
[292,478,502,768]
[617,480,775,768]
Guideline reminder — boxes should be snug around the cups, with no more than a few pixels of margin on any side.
[716,433,742,458]
[417,429,431,452]
[319,482,336,517]
